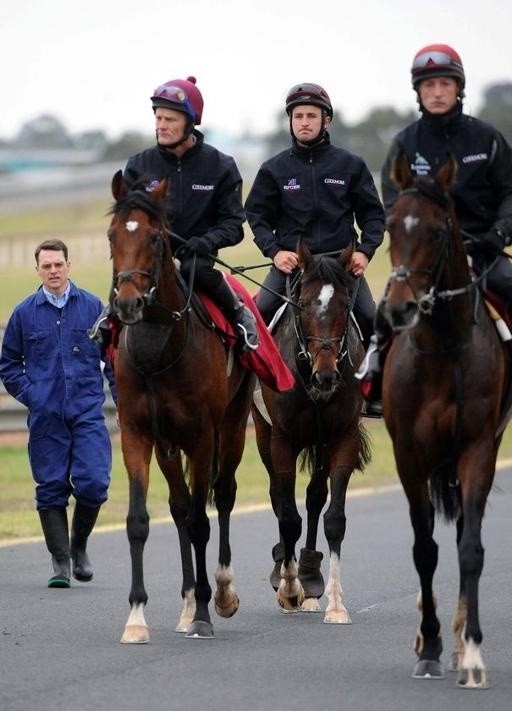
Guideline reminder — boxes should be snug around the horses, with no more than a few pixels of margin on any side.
[250,235,371,624]
[103,169,251,645]
[381,147,512,690]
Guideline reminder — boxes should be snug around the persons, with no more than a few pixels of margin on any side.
[366,45,512,415]
[0,241,119,588]
[124,76,258,357]
[244,83,386,354]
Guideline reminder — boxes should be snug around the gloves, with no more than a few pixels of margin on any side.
[481,229,505,251]
[183,237,212,254]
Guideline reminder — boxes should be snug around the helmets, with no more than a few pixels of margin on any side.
[412,44,465,83]
[286,83,332,121]
[150,76,203,125]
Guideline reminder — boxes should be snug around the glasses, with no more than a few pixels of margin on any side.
[154,86,196,123]
[412,52,463,68]
[286,84,328,102]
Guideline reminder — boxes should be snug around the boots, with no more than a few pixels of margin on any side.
[359,300,393,380]
[209,271,259,354]
[87,304,113,347]
[39,507,70,587]
[71,500,101,581]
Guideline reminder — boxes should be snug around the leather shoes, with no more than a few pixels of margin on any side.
[367,398,383,414]
